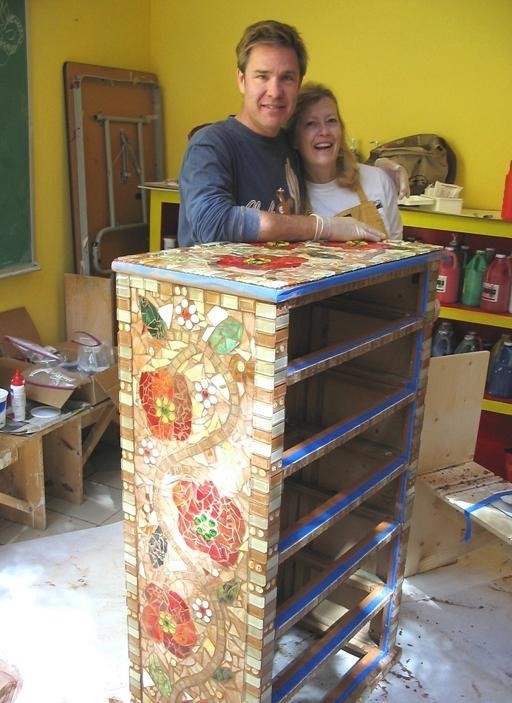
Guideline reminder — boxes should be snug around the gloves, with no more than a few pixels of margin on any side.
[310,213,387,242]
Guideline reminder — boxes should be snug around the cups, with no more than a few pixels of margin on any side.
[0,387,9,429]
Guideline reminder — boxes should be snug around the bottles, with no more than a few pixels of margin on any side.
[162,237,175,250]
[11,368,27,422]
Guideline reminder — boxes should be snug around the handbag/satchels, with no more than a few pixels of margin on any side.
[370,133,456,196]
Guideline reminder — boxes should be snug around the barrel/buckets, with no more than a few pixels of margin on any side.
[487,332,511,386]
[453,334,476,351]
[460,248,489,307]
[487,341,512,398]
[479,253,510,314]
[436,246,460,305]
[469,330,484,351]
[433,328,450,355]
[440,321,455,343]
[448,240,468,275]
[485,246,495,263]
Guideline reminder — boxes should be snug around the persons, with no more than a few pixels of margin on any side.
[178,18,410,248]
[282,80,404,245]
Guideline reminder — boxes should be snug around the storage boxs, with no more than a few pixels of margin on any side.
[0,306,121,410]
